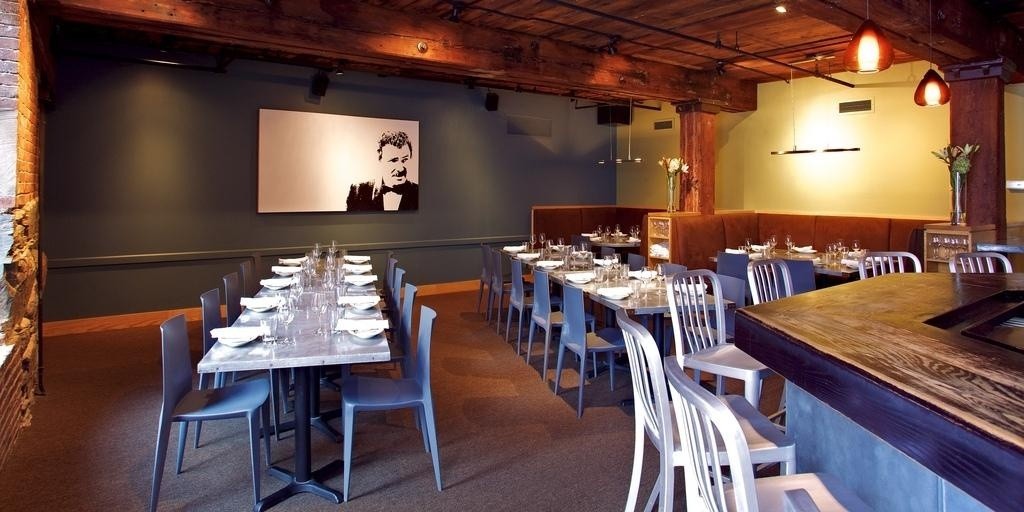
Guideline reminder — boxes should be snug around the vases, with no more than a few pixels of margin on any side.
[666,176,677,211]
[949,171,968,225]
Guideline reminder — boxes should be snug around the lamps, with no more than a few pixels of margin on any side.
[597,96,642,165]
[310,65,328,96]
[843,1,893,73]
[770,68,861,154]
[484,87,498,111]
[915,2,950,105]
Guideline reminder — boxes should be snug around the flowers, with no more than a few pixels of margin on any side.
[658,156,690,207]
[930,143,979,224]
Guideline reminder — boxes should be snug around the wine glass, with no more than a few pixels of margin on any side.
[926,233,972,263]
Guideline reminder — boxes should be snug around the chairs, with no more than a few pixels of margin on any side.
[149,314,271,512]
[774,257,816,297]
[478,244,512,322]
[489,248,528,335]
[746,258,795,306]
[196,254,390,512]
[378,249,399,330]
[355,283,420,430]
[858,251,922,279]
[949,252,1012,273]
[494,246,736,406]
[342,306,443,502]
[194,289,280,442]
[379,268,407,371]
[715,251,750,280]
[554,281,626,418]
[710,272,745,334]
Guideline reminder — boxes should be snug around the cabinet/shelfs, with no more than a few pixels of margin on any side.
[647,212,701,270]
[922,223,997,273]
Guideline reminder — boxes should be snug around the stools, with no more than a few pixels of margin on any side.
[667,268,784,427]
[616,308,797,512]
[664,354,875,512]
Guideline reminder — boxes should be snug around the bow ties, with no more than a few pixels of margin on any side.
[382,184,404,195]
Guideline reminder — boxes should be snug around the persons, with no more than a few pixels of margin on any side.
[345,128,418,211]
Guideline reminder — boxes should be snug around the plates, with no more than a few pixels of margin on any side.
[737,233,885,274]
[503,221,708,304]
[214,239,387,348]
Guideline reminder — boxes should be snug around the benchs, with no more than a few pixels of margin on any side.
[530,204,667,267]
[674,212,950,286]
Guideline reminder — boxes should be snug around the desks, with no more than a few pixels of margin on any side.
[709,245,897,279]
